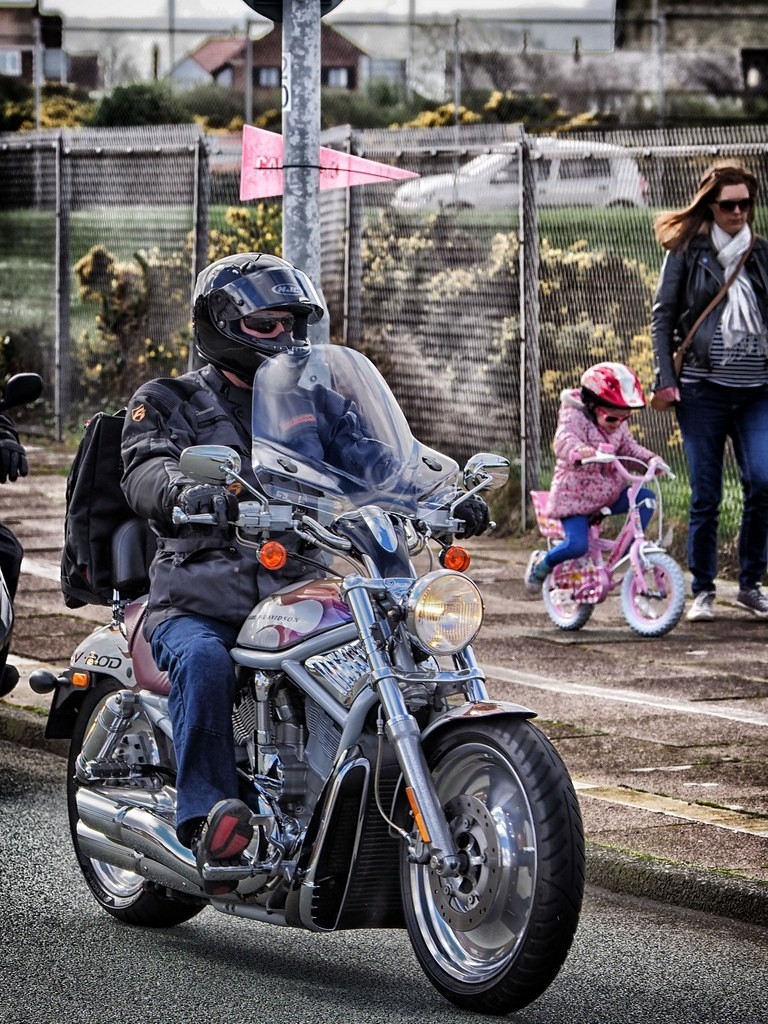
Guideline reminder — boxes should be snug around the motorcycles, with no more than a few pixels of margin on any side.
[22,340,586,1022]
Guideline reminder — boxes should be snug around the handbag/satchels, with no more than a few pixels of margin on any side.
[648,345,685,410]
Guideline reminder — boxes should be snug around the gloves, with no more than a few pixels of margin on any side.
[175,480,240,542]
[442,485,490,540]
[0,421,29,485]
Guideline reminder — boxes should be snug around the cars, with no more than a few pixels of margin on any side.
[385,136,653,225]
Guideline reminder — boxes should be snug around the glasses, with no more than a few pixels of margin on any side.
[714,198,752,212]
[593,405,633,424]
[242,313,296,335]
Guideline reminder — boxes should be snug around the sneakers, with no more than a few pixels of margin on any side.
[191,797,254,896]
[524,550,554,595]
[686,590,717,622]
[735,580,768,618]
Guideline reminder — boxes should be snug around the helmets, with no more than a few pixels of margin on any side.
[579,361,646,410]
[190,252,324,384]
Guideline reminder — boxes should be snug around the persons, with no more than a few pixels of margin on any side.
[652,165,768,621]
[121,253,491,897]
[0,392,28,695]
[525,362,672,596]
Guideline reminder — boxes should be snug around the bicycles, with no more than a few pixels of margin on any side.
[528,443,689,638]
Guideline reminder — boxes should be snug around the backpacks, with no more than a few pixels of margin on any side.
[59,378,257,609]
